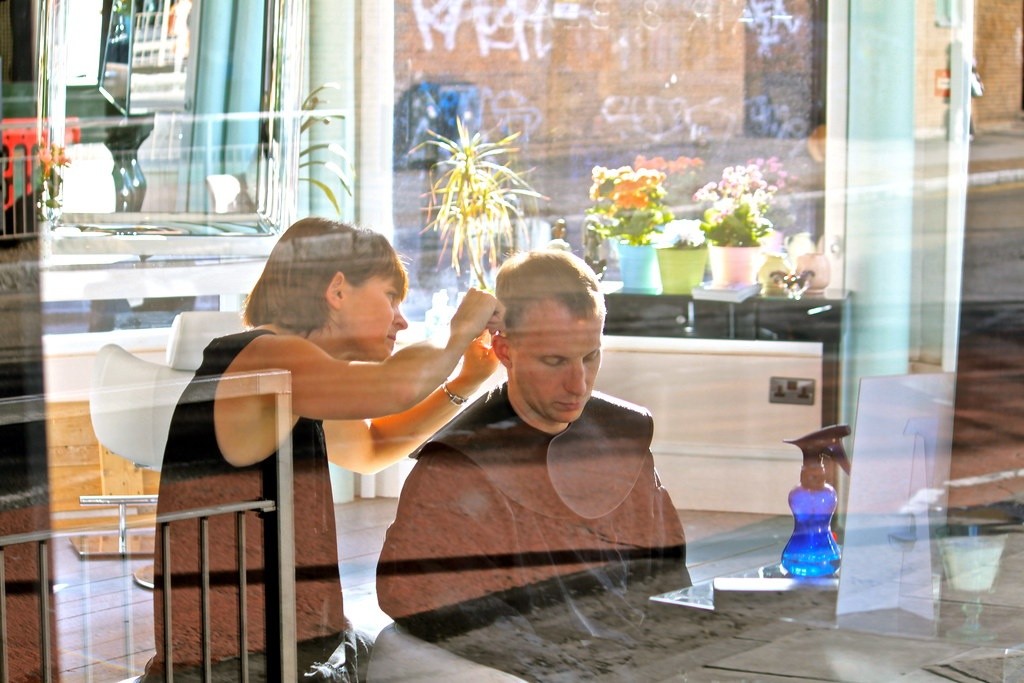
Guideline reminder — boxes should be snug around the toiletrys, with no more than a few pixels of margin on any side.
[781,423,854,582]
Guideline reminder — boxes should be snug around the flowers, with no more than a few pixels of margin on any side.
[691,163,777,246]
[585,163,675,245]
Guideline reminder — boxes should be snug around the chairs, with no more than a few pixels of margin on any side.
[78,306,253,590]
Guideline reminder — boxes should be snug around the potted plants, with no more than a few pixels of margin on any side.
[407,114,544,295]
[655,218,710,295]
[617,241,658,295]
[708,241,764,292]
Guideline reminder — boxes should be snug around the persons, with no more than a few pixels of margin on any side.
[136,217,505,683]
[376,247,695,683]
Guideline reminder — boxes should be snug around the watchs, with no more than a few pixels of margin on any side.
[442,383,468,406]
